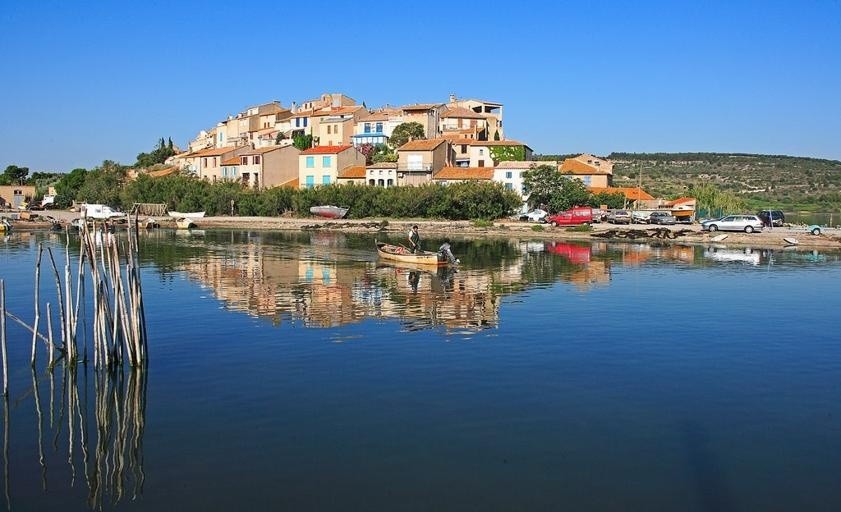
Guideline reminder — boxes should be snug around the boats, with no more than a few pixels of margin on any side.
[310,204,349,218]
[168,211,205,218]
[374,237,449,265]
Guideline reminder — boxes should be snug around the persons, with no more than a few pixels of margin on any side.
[408,226,422,253]
[408,271,421,295]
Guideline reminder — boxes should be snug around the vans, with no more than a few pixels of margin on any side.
[546,242,591,263]
[548,207,593,227]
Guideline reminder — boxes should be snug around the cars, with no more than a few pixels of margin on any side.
[702,210,785,232]
[592,208,675,225]
[703,247,761,265]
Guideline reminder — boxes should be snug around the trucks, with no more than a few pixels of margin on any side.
[81,204,126,221]
